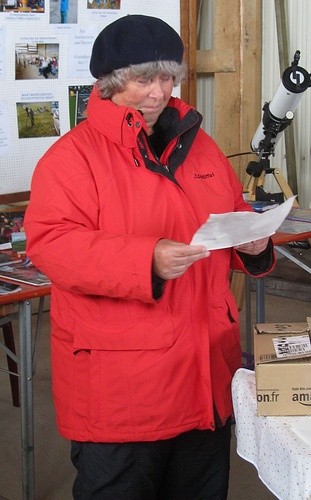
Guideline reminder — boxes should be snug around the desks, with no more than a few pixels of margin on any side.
[0,249,51,500]
[231,366,310,500]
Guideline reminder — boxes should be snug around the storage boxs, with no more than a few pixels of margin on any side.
[253,322,311,416]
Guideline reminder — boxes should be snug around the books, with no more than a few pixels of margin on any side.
[245,203,281,212]
[0,252,51,295]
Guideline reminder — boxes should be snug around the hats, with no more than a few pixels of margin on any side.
[89,15,184,80]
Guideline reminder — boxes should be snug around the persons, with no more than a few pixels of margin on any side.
[24,15,276,500]
